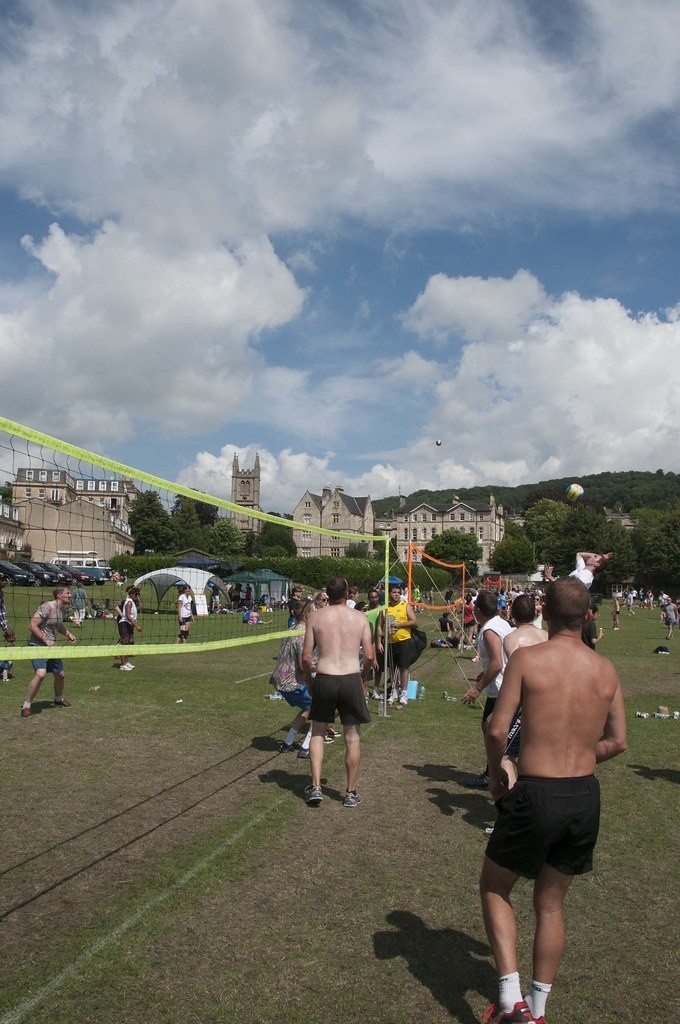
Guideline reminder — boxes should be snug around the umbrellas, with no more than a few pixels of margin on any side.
[381,576,402,584]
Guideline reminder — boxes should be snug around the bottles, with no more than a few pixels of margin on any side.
[377,700,384,716]
[418,684,426,700]
[654,712,669,719]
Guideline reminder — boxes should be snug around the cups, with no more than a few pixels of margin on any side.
[635,711,649,718]
[672,711,679,719]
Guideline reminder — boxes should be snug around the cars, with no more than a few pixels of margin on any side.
[30,560,73,586]
[57,563,96,587]
[10,561,61,587]
[0,561,35,587]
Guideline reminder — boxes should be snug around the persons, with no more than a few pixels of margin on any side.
[71,583,110,627]
[210,582,252,610]
[430,587,543,648]
[176,584,194,644]
[478,576,629,1024]
[270,577,435,807]
[244,607,273,625]
[460,589,603,838]
[544,552,613,590]
[21,585,77,719]
[115,585,142,671]
[259,594,264,605]
[610,587,680,640]
[0,572,16,682]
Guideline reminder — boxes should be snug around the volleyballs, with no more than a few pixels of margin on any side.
[565,483,585,503]
[436,440,442,446]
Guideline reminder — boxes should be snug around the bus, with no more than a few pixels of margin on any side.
[50,550,112,586]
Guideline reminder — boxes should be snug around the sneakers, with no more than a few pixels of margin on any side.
[482,1001,545,1023]
[343,792,361,807]
[20,706,32,719]
[465,776,490,788]
[54,699,73,708]
[125,662,135,669]
[119,665,132,671]
[297,747,309,757]
[306,785,323,801]
[279,740,302,753]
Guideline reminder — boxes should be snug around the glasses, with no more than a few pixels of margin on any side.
[321,598,329,602]
[295,591,301,593]
[134,592,140,596]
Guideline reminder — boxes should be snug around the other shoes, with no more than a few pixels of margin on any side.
[614,628,619,631]
[399,695,408,705]
[666,637,671,640]
[372,688,379,699]
[327,726,341,737]
[472,656,480,662]
[324,736,334,743]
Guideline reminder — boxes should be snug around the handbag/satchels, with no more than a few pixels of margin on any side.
[410,627,427,664]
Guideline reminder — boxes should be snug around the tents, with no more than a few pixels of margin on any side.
[134,566,232,610]
[178,556,219,566]
[226,570,291,601]
